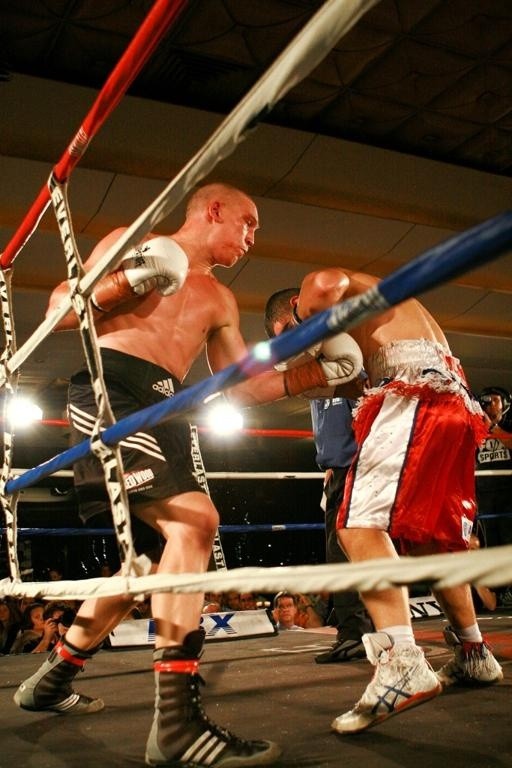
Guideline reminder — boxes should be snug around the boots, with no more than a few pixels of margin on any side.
[144,628,283,766]
[10,634,107,714]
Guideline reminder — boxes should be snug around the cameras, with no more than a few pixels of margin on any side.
[56,607,77,628]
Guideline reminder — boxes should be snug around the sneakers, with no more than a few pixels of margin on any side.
[315,637,369,663]
[331,632,443,737]
[433,629,503,684]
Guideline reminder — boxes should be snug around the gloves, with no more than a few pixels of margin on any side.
[91,236,189,311]
[286,331,364,401]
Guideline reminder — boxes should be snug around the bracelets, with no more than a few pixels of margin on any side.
[489,423,499,433]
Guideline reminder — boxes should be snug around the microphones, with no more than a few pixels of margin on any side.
[501,406,510,414]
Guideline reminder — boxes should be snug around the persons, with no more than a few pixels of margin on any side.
[472,386,512,547]
[466,532,498,612]
[14,181,364,767]
[264,268,504,734]
[310,369,378,663]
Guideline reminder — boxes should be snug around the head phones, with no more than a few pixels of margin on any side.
[480,386,512,408]
